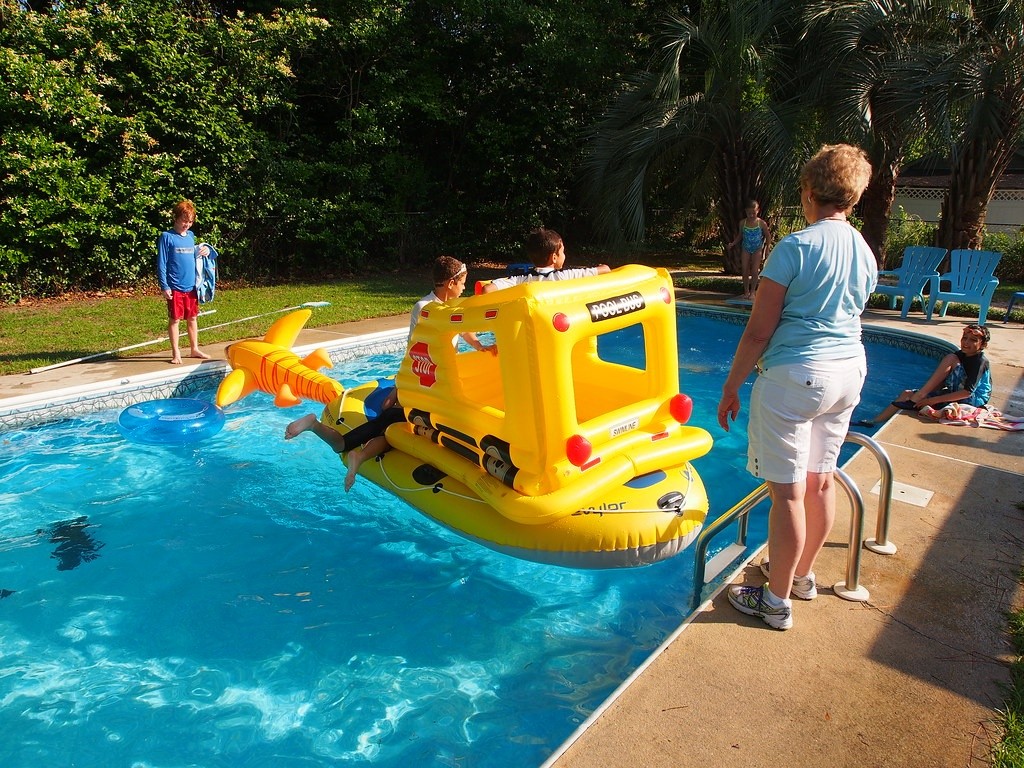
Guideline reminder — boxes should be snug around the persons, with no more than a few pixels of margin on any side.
[725,199,772,300]
[157,199,212,364]
[715,141,878,632]
[849,324,993,428]
[479,228,611,296]
[406,256,500,356]
[282,386,407,493]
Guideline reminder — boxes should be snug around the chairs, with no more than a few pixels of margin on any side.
[873,246,948,320]
[924,249,1003,327]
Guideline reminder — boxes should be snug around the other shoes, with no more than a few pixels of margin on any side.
[891,400,922,411]
[849,419,874,428]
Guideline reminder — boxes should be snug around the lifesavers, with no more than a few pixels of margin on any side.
[116,397,226,446]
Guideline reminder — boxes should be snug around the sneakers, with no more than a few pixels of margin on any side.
[760,558,817,600]
[728,582,793,629]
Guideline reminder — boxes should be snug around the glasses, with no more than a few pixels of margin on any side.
[963,328,985,338]
[435,264,467,284]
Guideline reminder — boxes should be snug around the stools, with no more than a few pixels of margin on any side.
[1004,292,1024,324]
